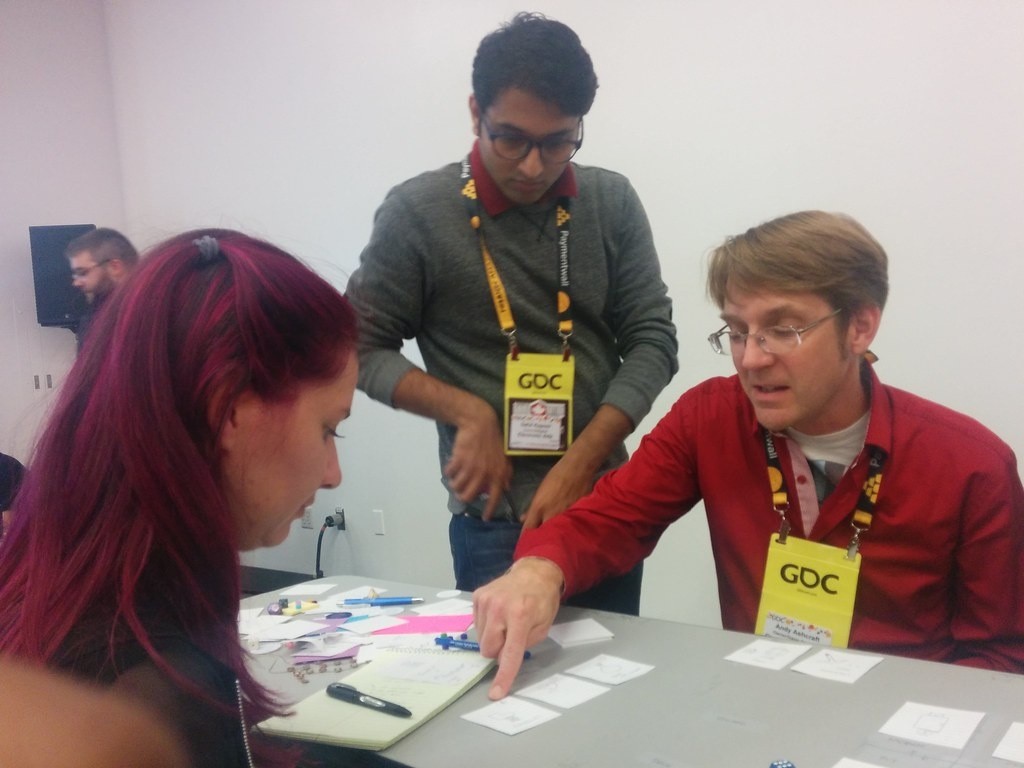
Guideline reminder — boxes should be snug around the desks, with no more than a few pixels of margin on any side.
[238,574,1024,768]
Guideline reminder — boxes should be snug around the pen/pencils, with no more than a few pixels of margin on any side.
[347,612,381,622]
[436,633,532,660]
[335,597,426,607]
[326,682,412,718]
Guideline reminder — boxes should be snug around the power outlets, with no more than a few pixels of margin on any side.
[335,507,346,530]
[301,506,313,529]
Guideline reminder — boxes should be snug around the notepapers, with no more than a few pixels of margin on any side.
[281,599,320,618]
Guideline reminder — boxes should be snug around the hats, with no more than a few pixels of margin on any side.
[0,451,26,512]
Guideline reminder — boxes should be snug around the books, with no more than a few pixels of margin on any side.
[248,650,491,750]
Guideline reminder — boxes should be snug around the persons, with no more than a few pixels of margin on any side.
[340,8,679,616]
[471,210,1023,701]
[0,225,357,768]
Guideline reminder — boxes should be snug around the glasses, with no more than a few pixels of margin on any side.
[481,112,583,165]
[708,308,842,355]
[70,257,120,281]
